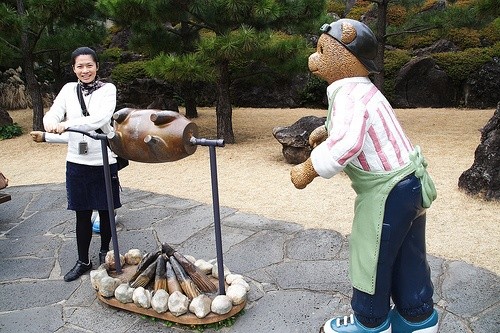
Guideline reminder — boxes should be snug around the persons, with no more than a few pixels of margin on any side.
[43,47,122,282]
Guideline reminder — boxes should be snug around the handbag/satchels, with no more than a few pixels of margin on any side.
[116,157,129,171]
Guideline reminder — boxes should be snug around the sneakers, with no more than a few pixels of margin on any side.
[64,260,92,282]
[99,248,110,266]
